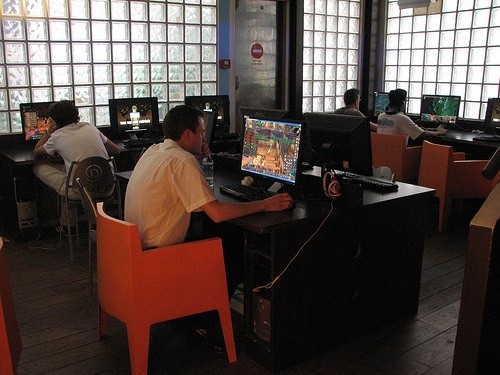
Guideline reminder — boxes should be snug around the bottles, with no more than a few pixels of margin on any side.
[200,153,214,190]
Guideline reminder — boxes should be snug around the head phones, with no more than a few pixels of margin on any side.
[321,172,342,200]
[384,99,406,115]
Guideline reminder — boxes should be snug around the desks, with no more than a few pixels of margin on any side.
[0,138,240,245]
[114,166,436,372]
[420,130,500,147]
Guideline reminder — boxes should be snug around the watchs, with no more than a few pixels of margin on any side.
[46,129,51,138]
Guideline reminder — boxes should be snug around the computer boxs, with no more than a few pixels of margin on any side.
[14,176,39,228]
[250,245,274,344]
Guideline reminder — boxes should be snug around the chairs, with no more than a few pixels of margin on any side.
[371,132,422,182]
[0,237,23,375]
[60,156,122,262]
[418,140,500,375]
[95,202,237,375]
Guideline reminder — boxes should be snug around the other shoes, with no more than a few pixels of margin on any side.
[50,224,78,237]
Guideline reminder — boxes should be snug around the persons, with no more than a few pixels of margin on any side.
[333,88,379,133]
[375,89,447,154]
[123,104,298,359]
[32,100,121,238]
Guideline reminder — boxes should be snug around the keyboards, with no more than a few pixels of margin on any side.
[473,136,500,144]
[124,140,164,147]
[341,172,399,194]
[219,183,273,203]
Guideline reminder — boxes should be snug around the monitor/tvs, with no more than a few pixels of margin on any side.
[20,101,75,142]
[239,117,305,194]
[239,107,291,139]
[303,113,373,197]
[109,97,160,142]
[484,98,500,127]
[420,95,461,132]
[185,94,230,130]
[373,92,406,118]
